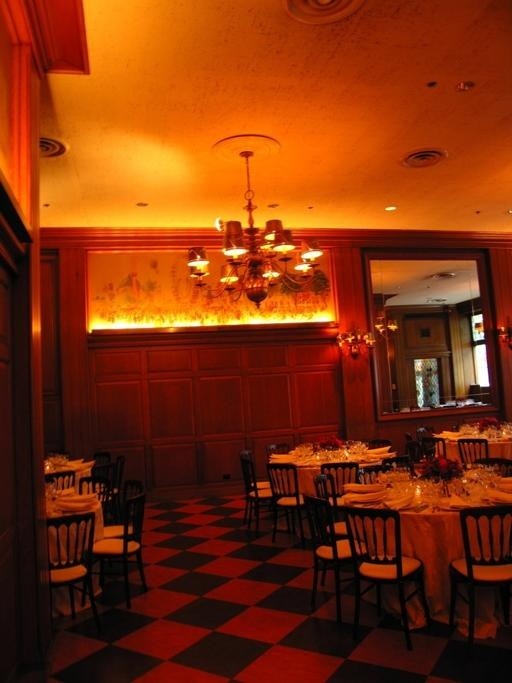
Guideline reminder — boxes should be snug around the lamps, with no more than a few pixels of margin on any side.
[374,308,399,338]
[501,327,506,339]
[335,326,375,358]
[187,135,324,309]
[476,322,483,332]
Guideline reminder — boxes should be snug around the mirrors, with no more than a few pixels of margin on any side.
[369,260,494,416]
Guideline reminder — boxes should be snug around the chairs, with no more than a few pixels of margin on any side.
[92,488,147,608]
[42,449,144,566]
[46,512,102,636]
